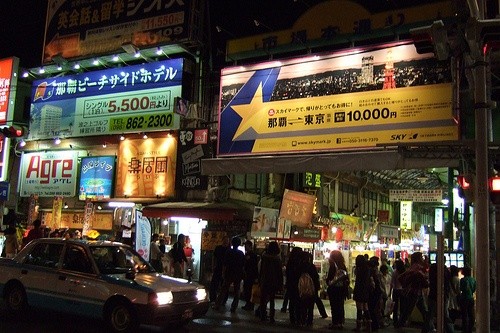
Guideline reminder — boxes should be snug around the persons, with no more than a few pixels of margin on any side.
[1,219,190,280]
[211,236,477,333]
[270,62,452,102]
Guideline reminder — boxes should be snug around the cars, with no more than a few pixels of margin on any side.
[0,239,208,333]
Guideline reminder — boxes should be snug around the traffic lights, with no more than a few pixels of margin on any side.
[458,173,474,197]
[0,125,24,137]
[489,174,500,204]
